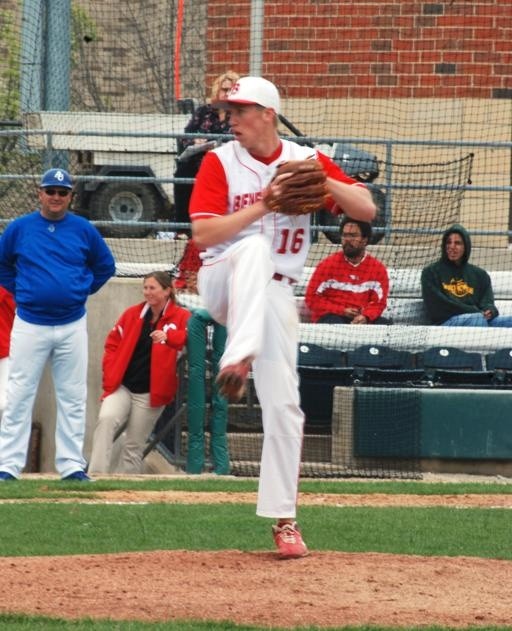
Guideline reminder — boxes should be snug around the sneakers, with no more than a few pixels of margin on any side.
[272,520,309,559]
[217,363,249,403]
[0,472,14,481]
[63,470,91,481]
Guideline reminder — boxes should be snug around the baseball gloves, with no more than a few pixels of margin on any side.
[263,160,328,213]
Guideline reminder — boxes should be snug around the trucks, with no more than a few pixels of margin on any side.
[23,93,388,249]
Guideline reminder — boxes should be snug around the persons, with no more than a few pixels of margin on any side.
[87,271,210,478]
[1,168,119,485]
[186,74,379,560]
[420,223,512,327]
[304,216,394,325]
[173,69,242,243]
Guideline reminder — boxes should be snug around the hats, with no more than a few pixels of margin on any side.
[212,76,281,115]
[39,168,73,189]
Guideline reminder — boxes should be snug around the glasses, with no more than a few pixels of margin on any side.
[45,189,71,197]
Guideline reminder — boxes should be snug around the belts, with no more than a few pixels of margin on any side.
[273,272,296,287]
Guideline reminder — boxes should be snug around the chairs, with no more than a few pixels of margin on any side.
[178,266,512,458]
